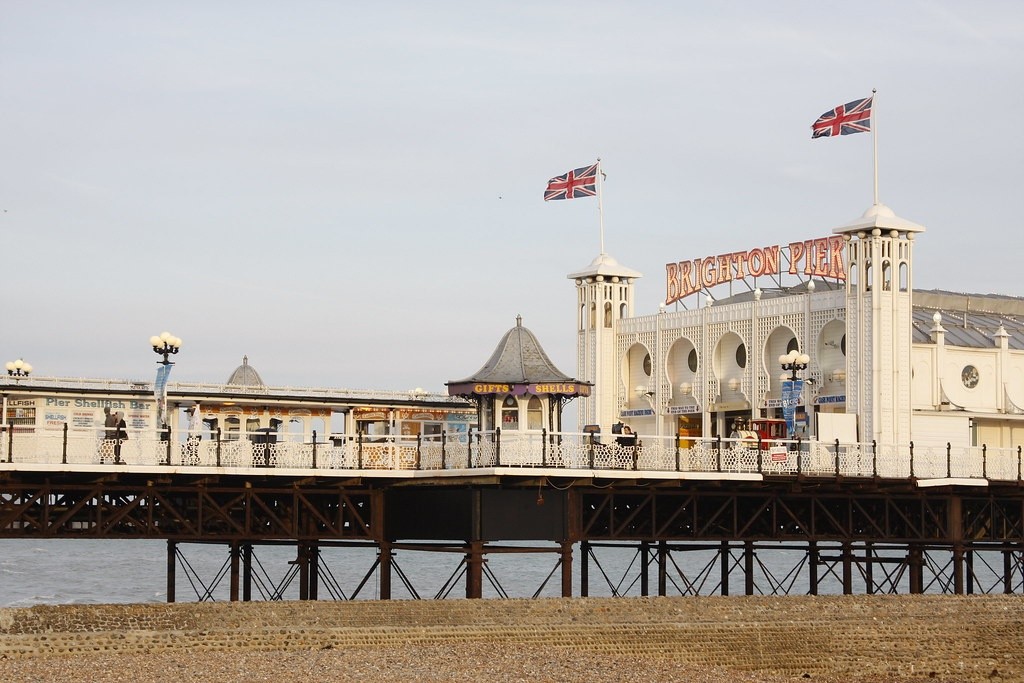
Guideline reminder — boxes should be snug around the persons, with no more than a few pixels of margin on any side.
[618,426,635,467]
[188,408,202,465]
[100,407,126,464]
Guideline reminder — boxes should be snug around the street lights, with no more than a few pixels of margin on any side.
[148,331,181,465]
[778,350,810,475]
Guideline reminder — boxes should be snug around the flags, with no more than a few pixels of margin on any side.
[544,164,598,201]
[811,96,873,138]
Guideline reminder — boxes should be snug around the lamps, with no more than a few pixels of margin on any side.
[779,372,790,385]
[728,379,741,395]
[680,382,694,399]
[833,369,845,387]
[635,385,648,403]
